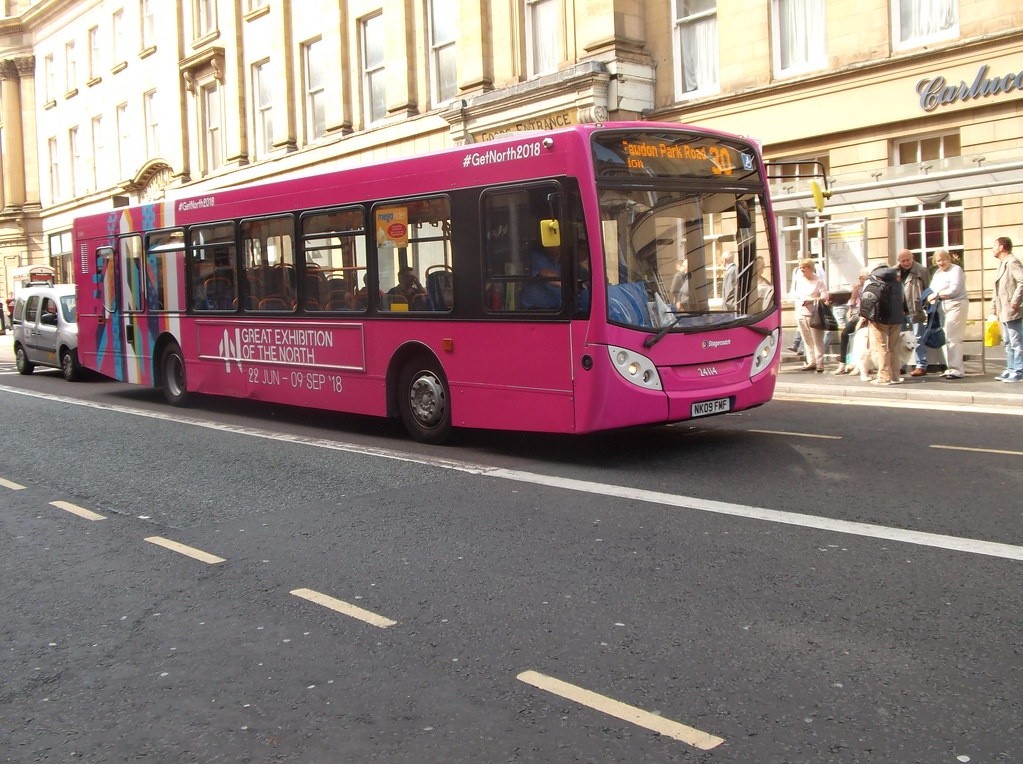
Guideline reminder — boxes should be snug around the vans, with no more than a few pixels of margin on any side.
[12,283,81,383]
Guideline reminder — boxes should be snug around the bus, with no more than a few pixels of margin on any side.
[72,115,832,446]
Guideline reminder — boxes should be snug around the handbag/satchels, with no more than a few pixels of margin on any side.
[985,317,1001,346]
[809,298,839,331]
[922,328,946,348]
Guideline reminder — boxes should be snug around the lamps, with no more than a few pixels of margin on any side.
[828,179,837,188]
[919,165,933,175]
[783,186,793,195]
[871,173,883,182]
[971,157,986,168]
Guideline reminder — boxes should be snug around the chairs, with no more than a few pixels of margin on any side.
[271,263,284,281]
[62,303,70,322]
[232,296,260,310]
[277,263,296,282]
[328,299,348,311]
[246,275,264,296]
[70,306,77,323]
[380,294,409,311]
[330,290,346,301]
[412,293,432,312]
[351,292,368,311]
[257,298,289,310]
[326,274,345,290]
[305,262,325,279]
[256,265,275,282]
[292,301,323,311]
[305,274,323,298]
[425,264,453,310]
[204,277,233,310]
[266,293,291,307]
[250,264,263,278]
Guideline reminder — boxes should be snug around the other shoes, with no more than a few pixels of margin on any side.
[900,370,907,374]
[850,369,859,375]
[818,368,824,372]
[788,346,797,352]
[911,369,926,376]
[835,367,844,375]
[1003,372,1023,382]
[995,371,1009,380]
[873,378,891,385]
[802,366,816,371]
[892,377,904,383]
[946,376,957,379]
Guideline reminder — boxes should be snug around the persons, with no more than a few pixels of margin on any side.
[988,237,1023,382]
[855,260,904,385]
[669,259,709,314]
[519,239,589,312]
[352,273,385,310]
[786,250,832,372]
[833,267,872,376]
[893,249,931,376]
[925,250,969,379]
[721,250,771,318]
[387,267,427,311]
[5,291,15,330]
